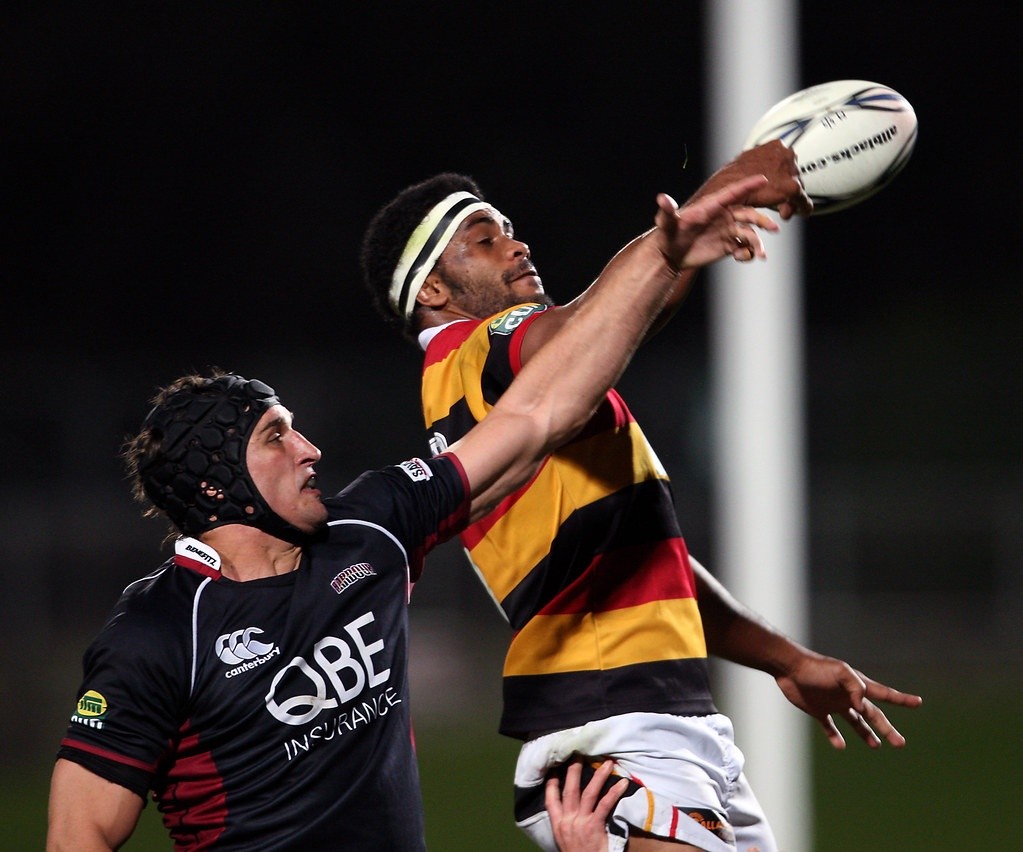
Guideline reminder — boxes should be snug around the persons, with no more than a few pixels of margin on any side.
[45,175,780,852]
[363,140,922,852]
[545,755,707,852]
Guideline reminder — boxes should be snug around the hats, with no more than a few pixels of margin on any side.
[139,372,275,538]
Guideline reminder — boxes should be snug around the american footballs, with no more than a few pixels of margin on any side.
[736,76,918,217]
